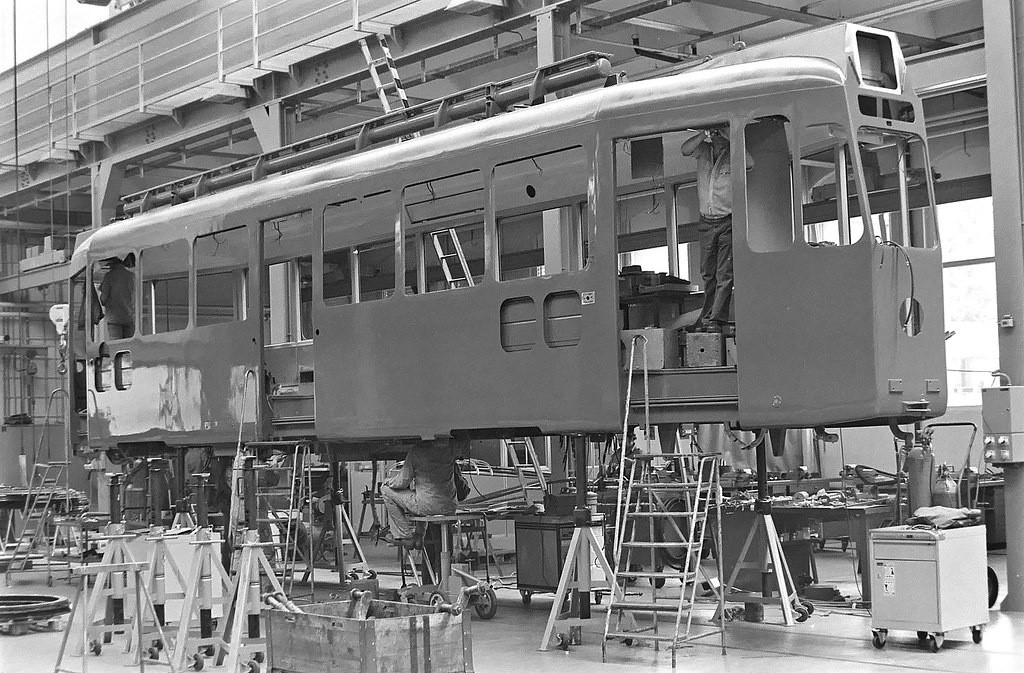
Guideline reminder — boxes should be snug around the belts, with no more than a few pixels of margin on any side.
[701,215,730,224]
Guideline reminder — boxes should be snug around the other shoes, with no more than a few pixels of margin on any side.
[707,321,724,333]
[685,320,708,332]
[386,534,415,551]
[412,532,424,550]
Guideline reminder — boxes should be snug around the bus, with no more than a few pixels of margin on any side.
[68,21,948,452]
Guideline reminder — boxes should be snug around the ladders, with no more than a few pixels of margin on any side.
[357,33,549,507]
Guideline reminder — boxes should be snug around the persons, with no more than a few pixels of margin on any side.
[378,441,471,584]
[681,127,754,332]
[98,259,136,340]
